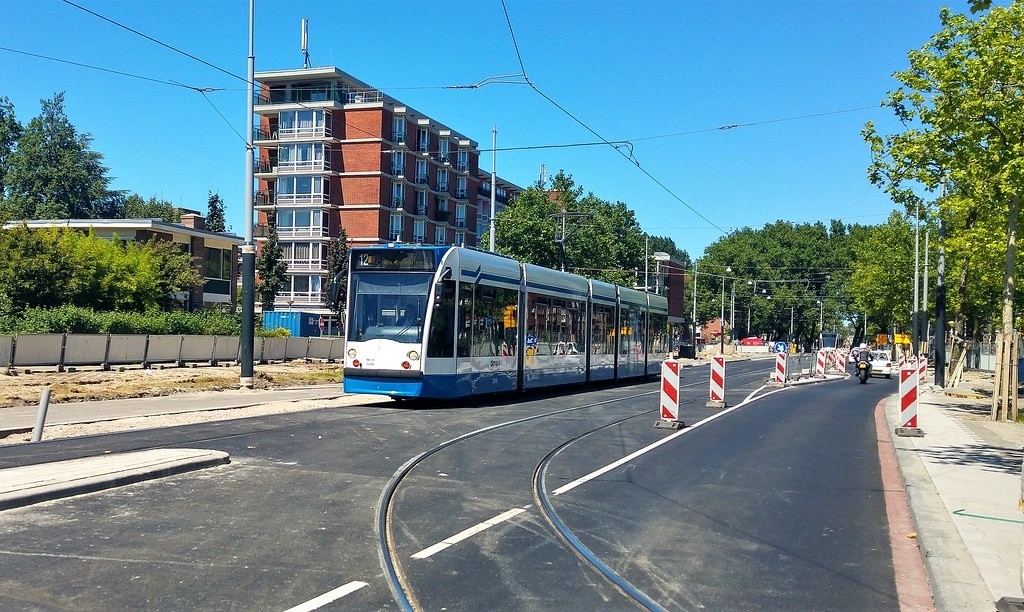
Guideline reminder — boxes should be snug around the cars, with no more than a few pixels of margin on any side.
[847,348,892,379]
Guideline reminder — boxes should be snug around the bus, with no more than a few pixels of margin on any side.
[328,211,669,405]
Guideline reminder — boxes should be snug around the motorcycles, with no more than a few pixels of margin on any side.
[853,357,875,385]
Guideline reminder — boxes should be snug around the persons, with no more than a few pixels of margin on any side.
[854,342,875,378]
[395,304,423,326]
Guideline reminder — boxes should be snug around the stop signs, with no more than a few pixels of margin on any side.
[319,317,327,333]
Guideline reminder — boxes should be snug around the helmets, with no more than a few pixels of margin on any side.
[859,343,866,349]
